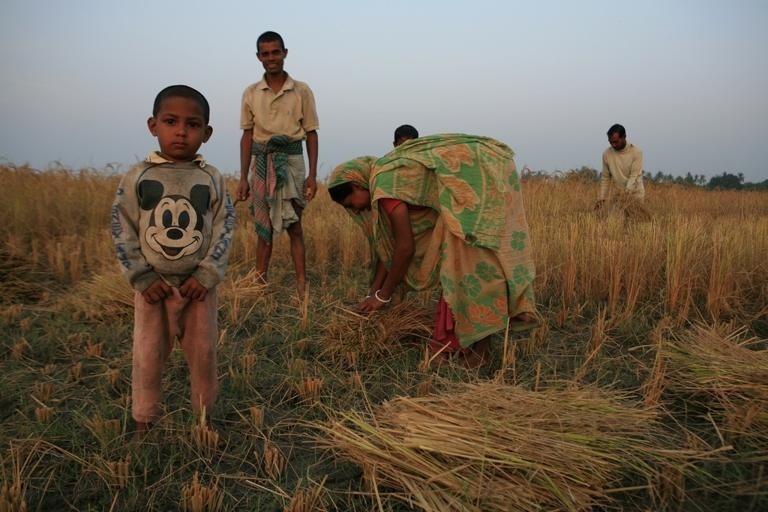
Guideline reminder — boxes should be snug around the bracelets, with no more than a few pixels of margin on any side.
[375,290,391,303]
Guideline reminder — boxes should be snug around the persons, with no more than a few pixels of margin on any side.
[109,85,235,442]
[594,124,644,211]
[328,134,536,369]
[236,32,319,300]
[393,125,418,147]
